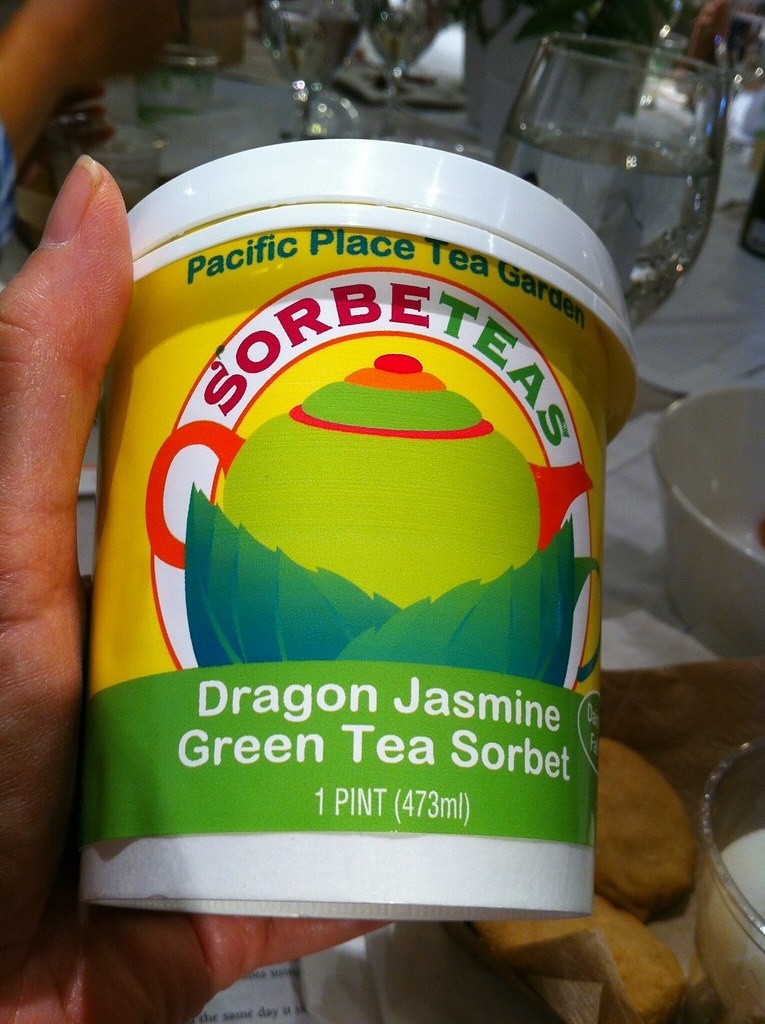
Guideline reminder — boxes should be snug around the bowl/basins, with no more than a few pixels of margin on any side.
[652,383,765,661]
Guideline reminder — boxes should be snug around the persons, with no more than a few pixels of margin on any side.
[0,155,399,1024]
[0,0,172,237]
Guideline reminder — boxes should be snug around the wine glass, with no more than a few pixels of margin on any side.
[252,0,448,138]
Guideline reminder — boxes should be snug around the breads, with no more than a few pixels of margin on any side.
[462,736,699,1024]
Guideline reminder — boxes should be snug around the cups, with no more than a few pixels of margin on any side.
[74,139,639,918]
[496,32,729,326]
[136,45,219,126]
[52,113,161,215]
[682,736,765,1024]
